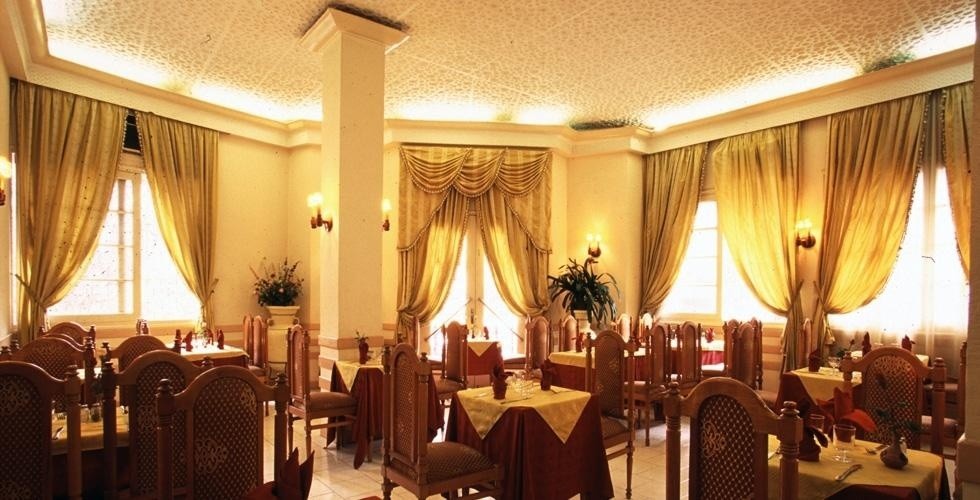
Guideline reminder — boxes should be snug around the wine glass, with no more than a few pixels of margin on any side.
[831,423,856,463]
[827,356,841,376]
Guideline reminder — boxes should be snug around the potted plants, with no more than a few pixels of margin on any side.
[542,257,625,338]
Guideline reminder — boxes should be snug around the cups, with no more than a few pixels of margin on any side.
[511,372,536,400]
[810,413,826,432]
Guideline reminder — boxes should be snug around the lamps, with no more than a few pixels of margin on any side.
[586,233,605,258]
[304,192,333,236]
[381,200,392,232]
[1,156,17,208]
[793,217,816,249]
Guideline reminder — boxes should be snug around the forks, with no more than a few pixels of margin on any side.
[53,413,66,440]
[864,445,887,455]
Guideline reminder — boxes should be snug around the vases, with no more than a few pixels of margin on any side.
[262,306,300,376]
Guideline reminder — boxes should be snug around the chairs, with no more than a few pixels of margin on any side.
[1,313,968,500]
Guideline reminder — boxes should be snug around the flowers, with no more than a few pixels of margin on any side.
[247,253,311,309]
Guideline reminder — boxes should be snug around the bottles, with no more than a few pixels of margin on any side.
[80,403,89,422]
[899,437,907,458]
[91,402,101,422]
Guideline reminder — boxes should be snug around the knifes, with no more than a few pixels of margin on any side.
[834,464,862,482]
